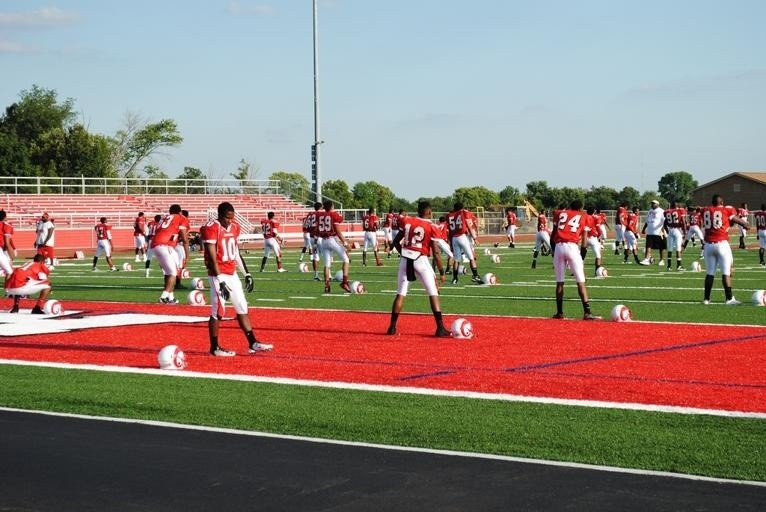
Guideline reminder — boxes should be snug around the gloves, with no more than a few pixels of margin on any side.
[220,281,232,300]
[244,275,254,293]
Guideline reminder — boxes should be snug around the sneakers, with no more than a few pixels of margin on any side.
[552,314,564,319]
[624,257,687,272]
[613,249,621,255]
[509,243,515,248]
[91,266,119,272]
[135,258,147,263]
[703,299,711,305]
[387,327,396,335]
[384,248,392,258]
[759,261,766,267]
[435,329,454,337]
[313,276,333,281]
[532,261,536,267]
[209,346,235,356]
[159,283,188,304]
[10,306,18,313]
[726,296,741,304]
[325,286,330,293]
[698,255,705,259]
[472,275,483,284]
[463,258,470,262]
[362,262,382,266]
[299,257,314,261]
[446,270,458,285]
[259,267,288,273]
[144,269,149,276]
[739,245,748,249]
[341,282,351,292]
[31,306,45,313]
[583,313,601,319]
[249,341,273,354]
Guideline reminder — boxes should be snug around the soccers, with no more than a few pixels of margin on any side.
[350,281,364,294]
[752,290,766,305]
[180,268,190,279]
[192,278,203,289]
[484,249,490,255]
[458,265,466,275]
[188,290,205,306]
[691,261,701,272]
[453,318,471,338]
[490,255,500,265]
[298,264,308,272]
[44,300,61,316]
[483,272,496,285]
[336,270,344,281]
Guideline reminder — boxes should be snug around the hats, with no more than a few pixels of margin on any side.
[650,200,659,204]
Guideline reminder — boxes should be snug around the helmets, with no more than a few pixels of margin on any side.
[691,261,702,272]
[752,290,766,307]
[181,269,189,279]
[484,248,490,254]
[336,271,345,281]
[188,290,209,305]
[351,280,364,293]
[44,300,64,316]
[484,273,496,284]
[490,255,500,263]
[451,319,473,339]
[457,265,467,274]
[299,263,309,272]
[192,277,204,289]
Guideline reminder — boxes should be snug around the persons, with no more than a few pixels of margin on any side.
[0,210,56,315]
[738,202,766,264]
[299,200,351,294]
[362,207,406,266]
[92,217,118,272]
[585,200,704,271]
[134,204,191,304]
[387,201,452,337]
[505,208,517,248]
[700,195,751,305]
[553,200,601,319]
[533,204,567,270]
[437,202,484,286]
[259,212,287,273]
[199,202,274,356]
